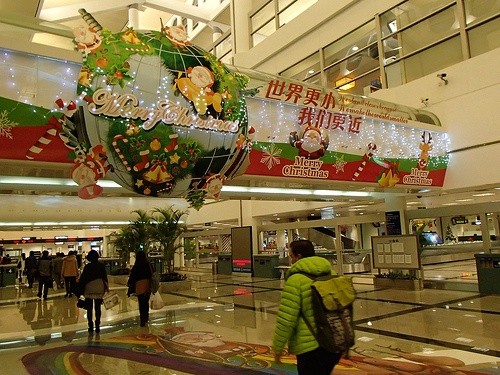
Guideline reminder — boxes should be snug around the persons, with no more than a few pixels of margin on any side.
[2,249,86,300]
[78,250,108,333]
[126,249,155,326]
[273,239,356,375]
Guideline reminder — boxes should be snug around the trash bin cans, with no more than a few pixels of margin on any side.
[212,260,218,274]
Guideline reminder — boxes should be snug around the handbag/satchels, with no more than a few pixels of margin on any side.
[104,292,121,310]
[151,291,164,309]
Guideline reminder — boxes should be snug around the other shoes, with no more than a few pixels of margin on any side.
[141,322,145,327]
[69,294,72,298]
[44,297,48,300]
[37,294,41,298]
[88,328,93,332]
[66,294,68,296]
[28,286,33,289]
[95,329,100,333]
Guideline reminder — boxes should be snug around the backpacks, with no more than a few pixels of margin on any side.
[288,272,356,354]
[147,262,160,294]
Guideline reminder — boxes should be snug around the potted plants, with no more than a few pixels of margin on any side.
[106,204,191,292]
[184,239,202,267]
[374,271,423,290]
